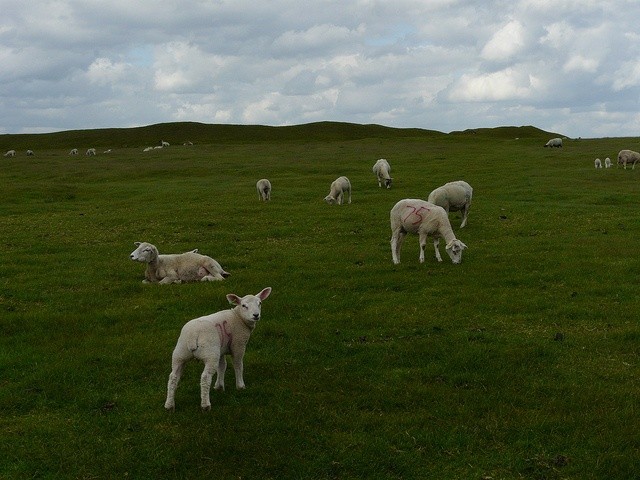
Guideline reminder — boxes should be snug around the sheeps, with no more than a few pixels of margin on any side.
[129,241,230,287]
[617,149,640,170]
[593,157,603,168]
[322,175,352,207]
[164,285,272,413]
[387,200,468,266]
[372,158,394,190]
[0,139,195,160]
[604,158,612,169]
[544,137,565,149]
[256,178,272,202]
[427,180,474,230]
